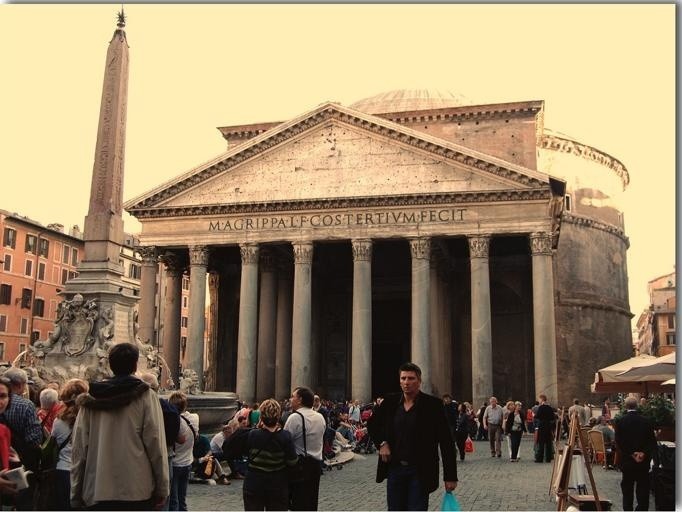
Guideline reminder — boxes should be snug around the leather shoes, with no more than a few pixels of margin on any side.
[232,472,245,479]
[221,478,231,485]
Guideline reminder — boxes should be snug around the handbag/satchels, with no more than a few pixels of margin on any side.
[40,432,71,470]
[294,411,320,484]
[464,435,474,452]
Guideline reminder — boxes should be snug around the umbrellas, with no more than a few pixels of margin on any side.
[590,352,675,401]
[616,350,675,378]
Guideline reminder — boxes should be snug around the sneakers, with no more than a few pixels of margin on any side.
[510,459,515,462]
[516,459,519,462]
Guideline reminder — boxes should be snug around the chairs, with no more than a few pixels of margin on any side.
[587,430,610,466]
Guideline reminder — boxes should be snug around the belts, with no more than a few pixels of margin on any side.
[392,460,415,467]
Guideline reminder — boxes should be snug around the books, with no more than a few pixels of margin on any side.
[4,464,34,490]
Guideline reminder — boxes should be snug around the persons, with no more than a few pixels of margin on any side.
[249,403,260,429]
[0,380,23,511]
[168,392,199,512]
[476,402,489,441]
[568,399,586,426]
[601,396,611,418]
[592,416,615,470]
[441,393,458,453]
[584,401,591,423]
[454,403,472,460]
[190,451,232,485]
[235,415,247,432]
[36,388,61,441]
[531,401,540,417]
[222,398,298,511]
[482,397,503,458]
[69,342,171,512]
[3,367,42,511]
[502,401,521,459]
[51,378,88,512]
[314,395,384,456]
[365,363,459,512]
[140,373,180,483]
[283,386,326,511]
[233,400,248,418]
[534,395,556,464]
[210,426,246,479]
[614,397,657,511]
[526,404,534,433]
[505,401,527,462]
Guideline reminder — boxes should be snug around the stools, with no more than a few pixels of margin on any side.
[558,447,583,495]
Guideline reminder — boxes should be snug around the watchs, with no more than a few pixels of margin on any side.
[378,440,388,447]
